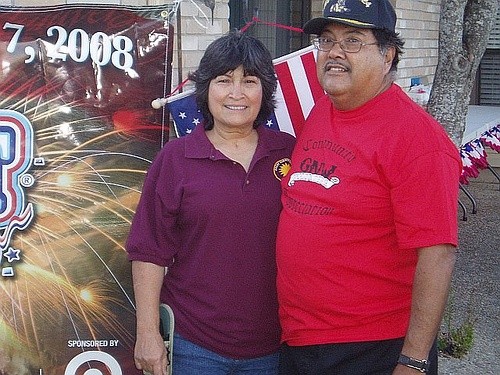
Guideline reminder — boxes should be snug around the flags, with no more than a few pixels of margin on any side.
[152,46,326,139]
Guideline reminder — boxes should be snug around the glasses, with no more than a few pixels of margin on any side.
[311,35,381,54]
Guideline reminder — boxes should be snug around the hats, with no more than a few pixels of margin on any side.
[302,0,398,34]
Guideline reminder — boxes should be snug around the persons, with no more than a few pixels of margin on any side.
[126,33,296,375]
[276,0,462,375]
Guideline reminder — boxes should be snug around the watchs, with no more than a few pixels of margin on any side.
[396,353,430,373]
